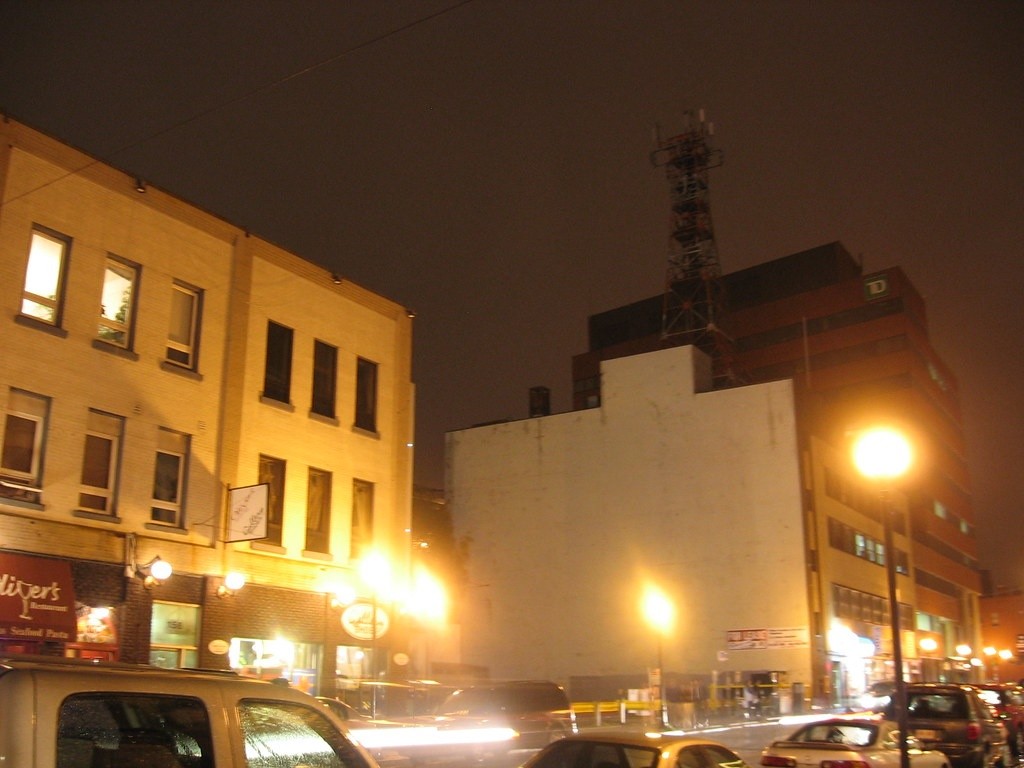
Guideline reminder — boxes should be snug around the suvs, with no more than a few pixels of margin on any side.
[882,682,1024,768]
[397,680,578,766]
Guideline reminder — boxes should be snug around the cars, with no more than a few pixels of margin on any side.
[518,732,751,768]
[760,718,952,768]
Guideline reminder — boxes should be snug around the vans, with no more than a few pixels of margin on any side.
[0,654,382,768]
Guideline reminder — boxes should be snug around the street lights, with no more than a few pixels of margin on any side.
[848,422,917,767]
[361,547,395,720]
[638,587,678,732]
